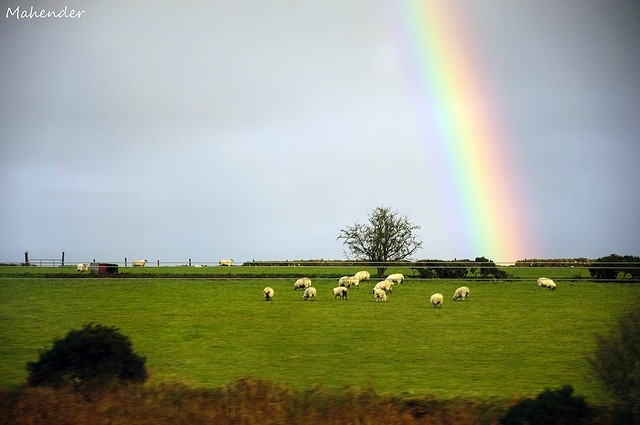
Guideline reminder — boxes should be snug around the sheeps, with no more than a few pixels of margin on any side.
[373,280,394,295]
[386,273,404,285]
[78,263,88,273]
[293,278,311,290]
[375,289,386,301]
[132,260,147,266]
[339,276,349,288]
[263,286,274,301]
[430,293,443,308]
[347,277,360,290]
[355,271,370,281]
[333,286,348,300]
[303,287,316,301]
[453,286,469,300]
[219,258,234,266]
[537,277,557,292]
